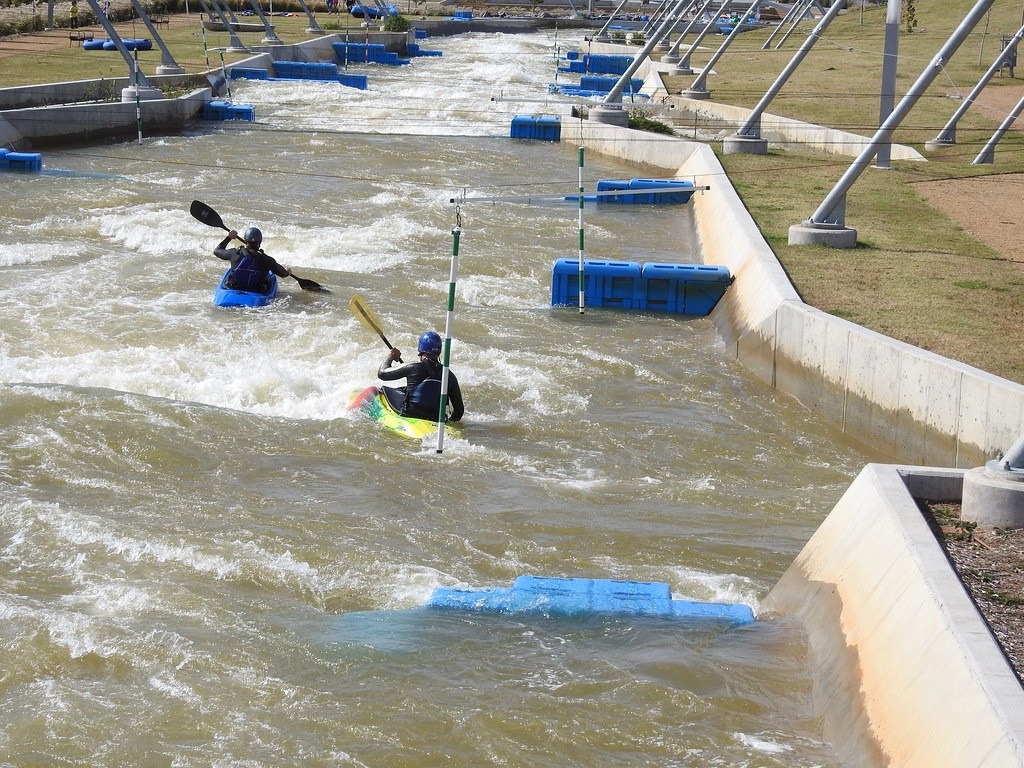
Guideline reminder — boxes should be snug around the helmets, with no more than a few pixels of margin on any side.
[418,332,442,354]
[244,227,262,243]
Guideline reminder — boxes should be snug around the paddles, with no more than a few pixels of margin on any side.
[190,199,331,294]
[349,294,405,366]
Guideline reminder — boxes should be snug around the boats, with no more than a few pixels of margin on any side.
[204,21,275,32]
[213,267,278,308]
[83,38,152,51]
[346,386,465,441]
[351,4,398,19]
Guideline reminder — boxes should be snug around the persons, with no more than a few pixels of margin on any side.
[213,225,292,297]
[378,332,464,423]
[346,0,355,14]
[70,0,79,29]
[326,0,338,15]
[102,1,111,30]
